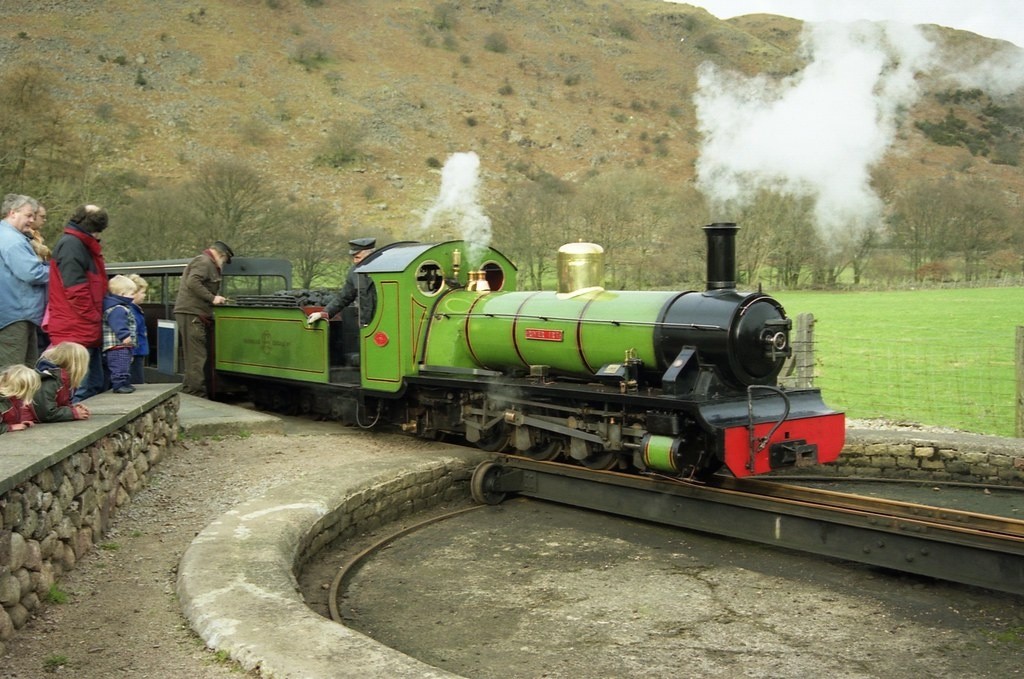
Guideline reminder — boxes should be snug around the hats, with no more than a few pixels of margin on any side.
[348,238,376,255]
[214,240,234,264]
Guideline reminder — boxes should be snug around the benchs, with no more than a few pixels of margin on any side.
[139,303,176,367]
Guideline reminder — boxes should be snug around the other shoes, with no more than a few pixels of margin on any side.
[113,385,135,394]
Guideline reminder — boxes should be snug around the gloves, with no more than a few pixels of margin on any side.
[307,312,321,324]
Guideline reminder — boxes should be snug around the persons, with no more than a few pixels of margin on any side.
[0,194,150,435]
[173,240,234,400]
[307,238,378,326]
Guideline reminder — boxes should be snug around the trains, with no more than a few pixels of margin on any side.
[104,221,849,492]
